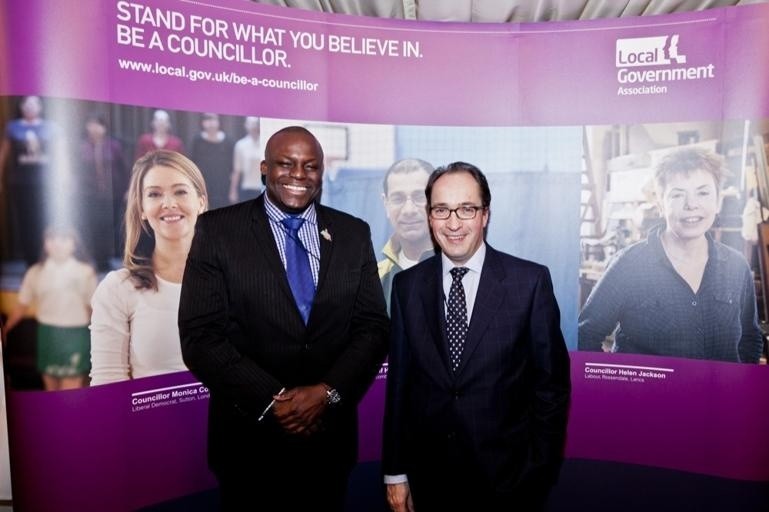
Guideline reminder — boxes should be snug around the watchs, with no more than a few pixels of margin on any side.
[324,385,342,406]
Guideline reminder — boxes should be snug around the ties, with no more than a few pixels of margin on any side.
[445,267,467,374]
[280,218,315,327]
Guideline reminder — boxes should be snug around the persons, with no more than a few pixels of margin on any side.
[374,153,441,320]
[71,110,132,276]
[576,144,765,364]
[228,114,264,203]
[133,107,184,167]
[181,124,392,512]
[87,148,210,391]
[0,223,100,393]
[380,162,574,512]
[0,93,70,275]
[190,112,235,206]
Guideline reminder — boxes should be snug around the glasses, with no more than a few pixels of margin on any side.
[428,207,484,220]
